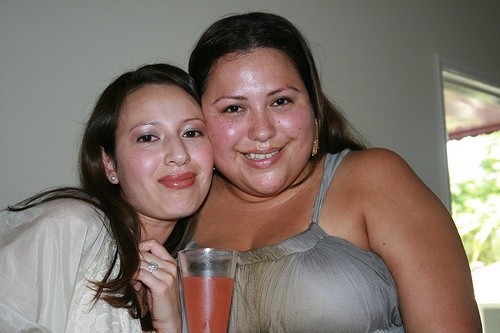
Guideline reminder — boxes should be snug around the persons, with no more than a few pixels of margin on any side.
[173,11,483,333]
[1,63,215,333]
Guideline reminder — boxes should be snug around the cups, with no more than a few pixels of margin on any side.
[178,248,238,333]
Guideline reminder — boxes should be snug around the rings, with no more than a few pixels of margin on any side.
[149,262,158,271]
